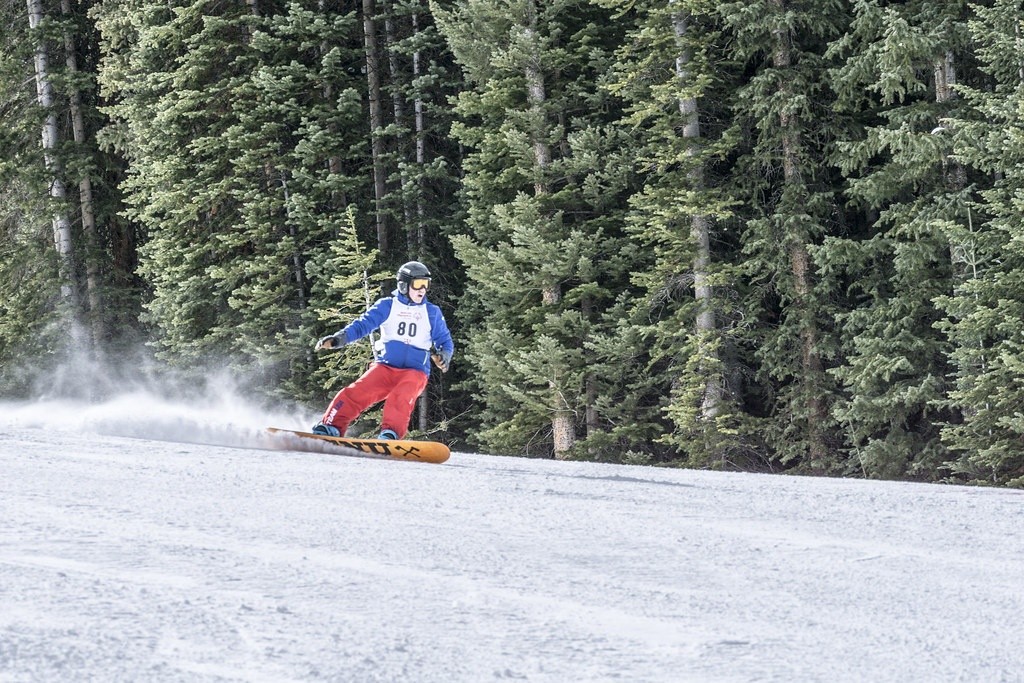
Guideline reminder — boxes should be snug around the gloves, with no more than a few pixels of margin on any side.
[315,335,338,350]
[429,353,450,373]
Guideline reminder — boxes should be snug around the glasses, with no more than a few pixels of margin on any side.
[411,279,430,290]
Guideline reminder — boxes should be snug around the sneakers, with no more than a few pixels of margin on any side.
[378,429,398,440]
[312,422,341,437]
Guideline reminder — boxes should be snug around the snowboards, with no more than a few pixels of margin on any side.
[264,426,451,464]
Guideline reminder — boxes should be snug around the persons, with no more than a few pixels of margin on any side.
[312,261,455,440]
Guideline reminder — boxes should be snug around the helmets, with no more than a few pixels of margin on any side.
[396,261,432,295]
